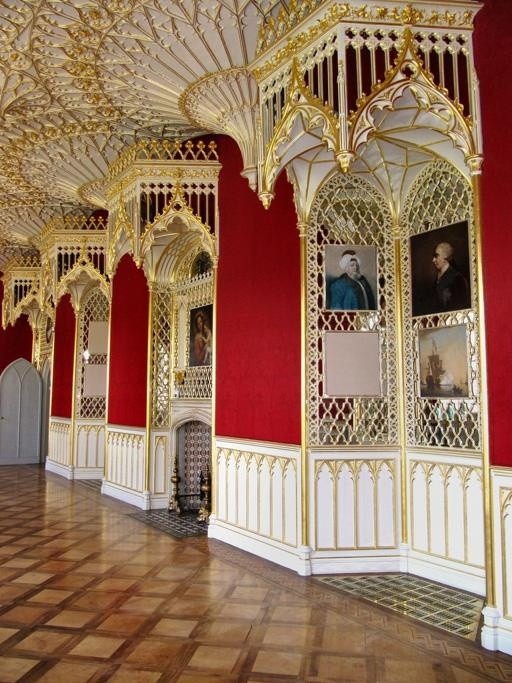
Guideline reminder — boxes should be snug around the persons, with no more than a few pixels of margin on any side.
[428,242,470,311]
[193,311,212,365]
[330,250,375,310]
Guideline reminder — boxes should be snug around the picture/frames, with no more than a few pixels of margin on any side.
[189,303,213,366]
[414,323,469,399]
[407,218,473,320]
[322,243,380,313]
[322,328,381,399]
